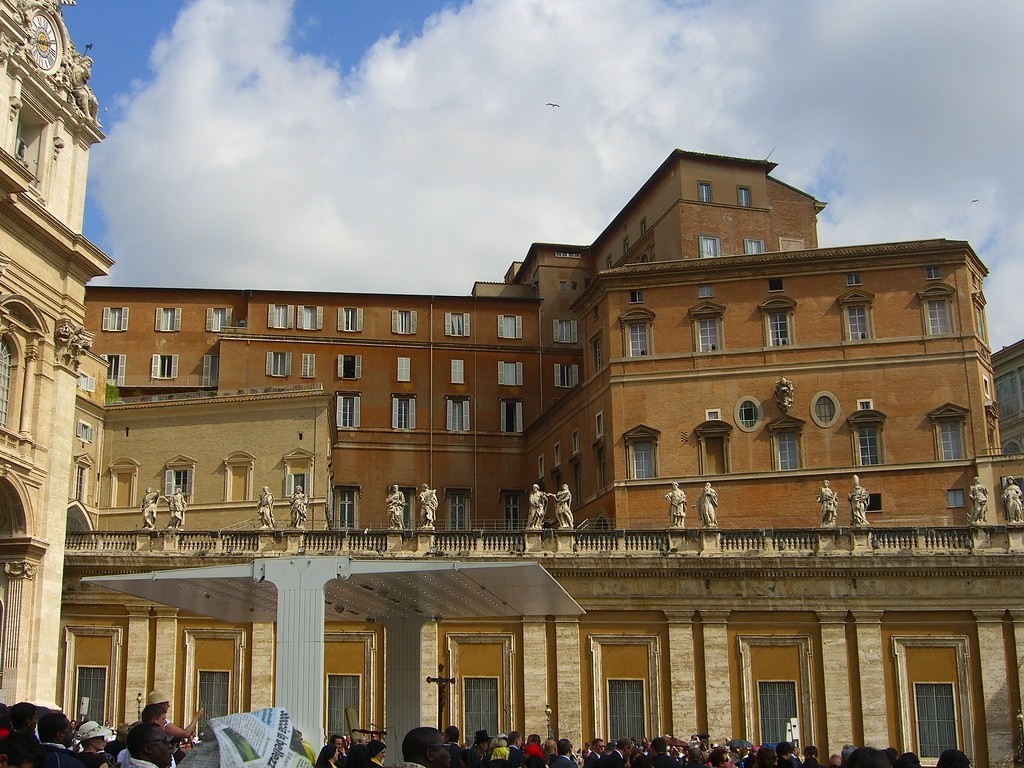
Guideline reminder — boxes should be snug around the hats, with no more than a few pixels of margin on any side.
[146,689,169,705]
[776,740,792,753]
[35,704,63,724]
[585,742,590,746]
[841,744,857,759]
[691,735,697,738]
[474,730,491,744]
[751,746,758,752]
[367,739,387,756]
[74,721,112,740]
[789,742,797,749]
[497,733,508,739]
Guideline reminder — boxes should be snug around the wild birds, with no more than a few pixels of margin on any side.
[970,200,979,204]
[546,103,560,108]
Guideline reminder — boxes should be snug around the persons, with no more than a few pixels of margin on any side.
[140,487,160,530]
[289,484,308,529]
[526,484,550,530]
[1000,474,1023,524]
[0,691,974,768]
[420,483,439,526]
[969,475,989,523]
[257,485,275,529]
[551,483,575,528]
[664,482,687,528]
[693,482,719,527]
[845,474,871,526]
[385,484,405,529]
[161,488,187,530]
[816,480,838,526]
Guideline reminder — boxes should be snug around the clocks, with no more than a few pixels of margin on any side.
[31,14,58,69]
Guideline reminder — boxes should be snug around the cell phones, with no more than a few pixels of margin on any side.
[201,702,203,710]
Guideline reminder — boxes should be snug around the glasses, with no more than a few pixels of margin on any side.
[148,737,170,746]
[96,736,108,742]
[431,743,451,750]
[598,745,606,748]
[379,751,385,754]
[61,728,77,734]
[165,700,170,705]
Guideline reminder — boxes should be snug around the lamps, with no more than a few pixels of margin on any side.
[84,43,92,56]
